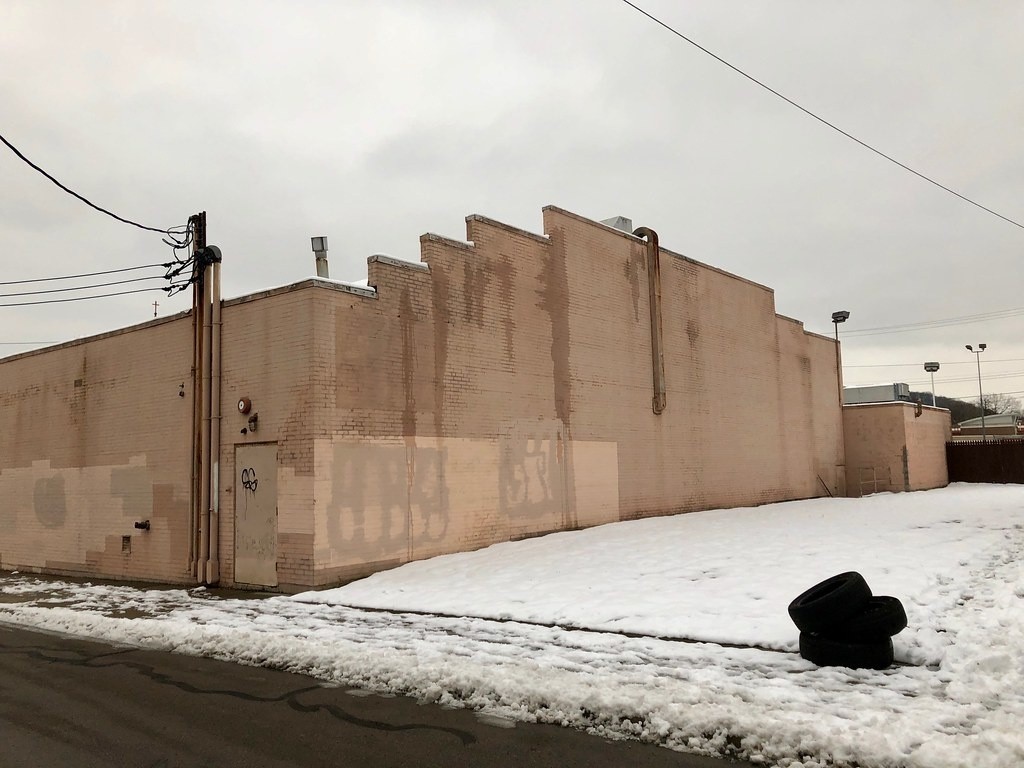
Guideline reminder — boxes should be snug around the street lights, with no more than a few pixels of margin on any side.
[965,344,987,441]
